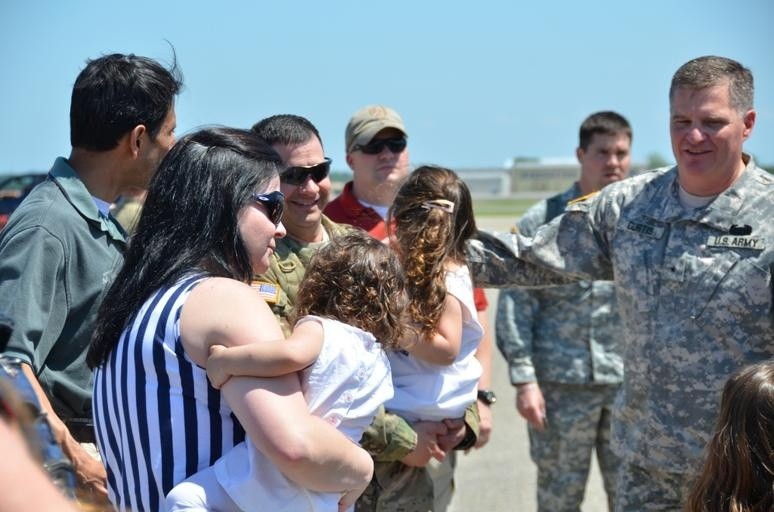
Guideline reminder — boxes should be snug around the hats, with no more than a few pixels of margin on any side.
[345,105,408,155]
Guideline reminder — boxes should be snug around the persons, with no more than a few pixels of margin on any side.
[492,110,632,511]
[87,125,375,510]
[163,234,404,511]
[680,356,774,512]
[0,424,77,512]
[459,56,773,512]
[0,53,177,512]
[372,163,483,423]
[251,114,480,511]
[318,105,495,511]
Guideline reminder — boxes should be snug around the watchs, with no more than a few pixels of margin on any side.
[477,390,494,408]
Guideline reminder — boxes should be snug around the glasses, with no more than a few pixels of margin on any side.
[275,156,333,187]
[245,189,286,225]
[357,135,409,157]
